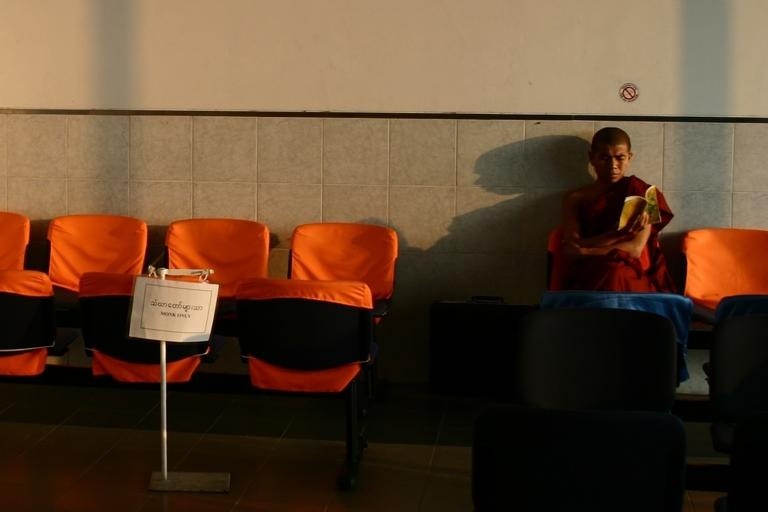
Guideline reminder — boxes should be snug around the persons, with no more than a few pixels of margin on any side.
[558,127,675,294]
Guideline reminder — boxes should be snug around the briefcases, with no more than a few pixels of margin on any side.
[427,295,540,398]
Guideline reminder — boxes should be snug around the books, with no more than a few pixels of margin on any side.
[617,183,664,230]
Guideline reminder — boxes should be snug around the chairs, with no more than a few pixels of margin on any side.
[0,212,400,491]
[472,227,768,512]
